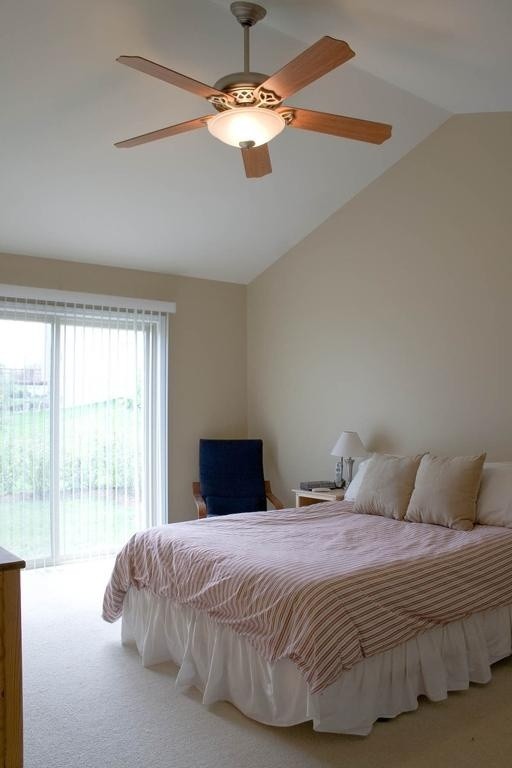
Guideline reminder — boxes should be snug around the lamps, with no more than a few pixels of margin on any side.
[207,2,285,151]
[330,430,371,488]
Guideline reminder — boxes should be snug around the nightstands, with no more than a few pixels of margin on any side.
[291,488,346,507]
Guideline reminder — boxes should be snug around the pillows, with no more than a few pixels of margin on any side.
[404,452,487,531]
[477,460,512,528]
[346,450,428,521]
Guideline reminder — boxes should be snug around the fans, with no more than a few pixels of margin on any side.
[111,0,392,181]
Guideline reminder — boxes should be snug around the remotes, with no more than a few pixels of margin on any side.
[312,487,330,492]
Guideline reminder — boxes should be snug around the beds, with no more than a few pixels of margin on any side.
[100,451,511,738]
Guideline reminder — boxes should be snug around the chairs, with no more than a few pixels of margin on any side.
[191,439,285,519]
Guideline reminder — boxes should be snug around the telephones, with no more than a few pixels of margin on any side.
[335,457,346,489]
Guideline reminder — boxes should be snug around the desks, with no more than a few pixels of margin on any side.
[0,546,27,725]
